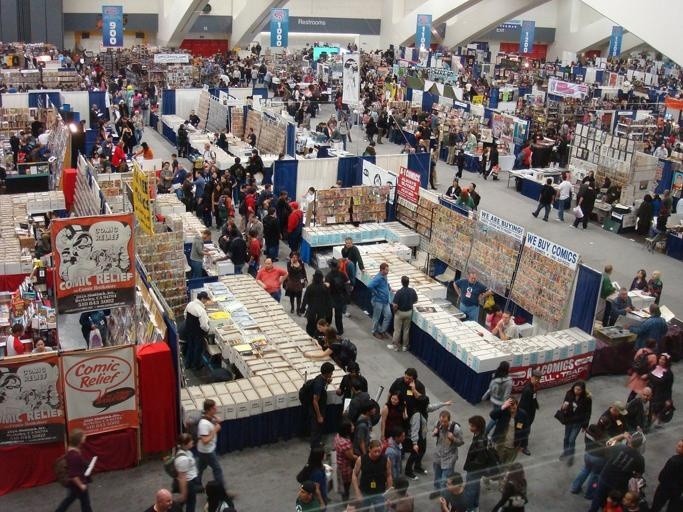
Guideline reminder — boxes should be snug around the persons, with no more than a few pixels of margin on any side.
[1,40,682,512]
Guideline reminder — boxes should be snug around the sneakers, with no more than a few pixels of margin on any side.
[387,344,399,351]
[373,331,383,340]
[403,345,411,351]
[405,471,419,480]
[414,466,428,474]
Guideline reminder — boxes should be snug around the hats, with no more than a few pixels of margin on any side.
[198,290,211,300]
[614,400,628,415]
[301,480,315,493]
[532,370,542,377]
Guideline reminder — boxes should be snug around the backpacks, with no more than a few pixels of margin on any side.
[632,348,656,375]
[51,456,73,488]
[484,438,502,481]
[163,449,186,478]
[298,376,321,409]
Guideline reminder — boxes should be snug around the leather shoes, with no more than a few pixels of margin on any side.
[522,448,530,455]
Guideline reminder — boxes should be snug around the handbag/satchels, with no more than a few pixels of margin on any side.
[507,496,528,512]
[554,410,568,424]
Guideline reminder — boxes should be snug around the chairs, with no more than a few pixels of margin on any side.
[433,266,456,291]
[640,232,662,253]
[203,337,221,372]
